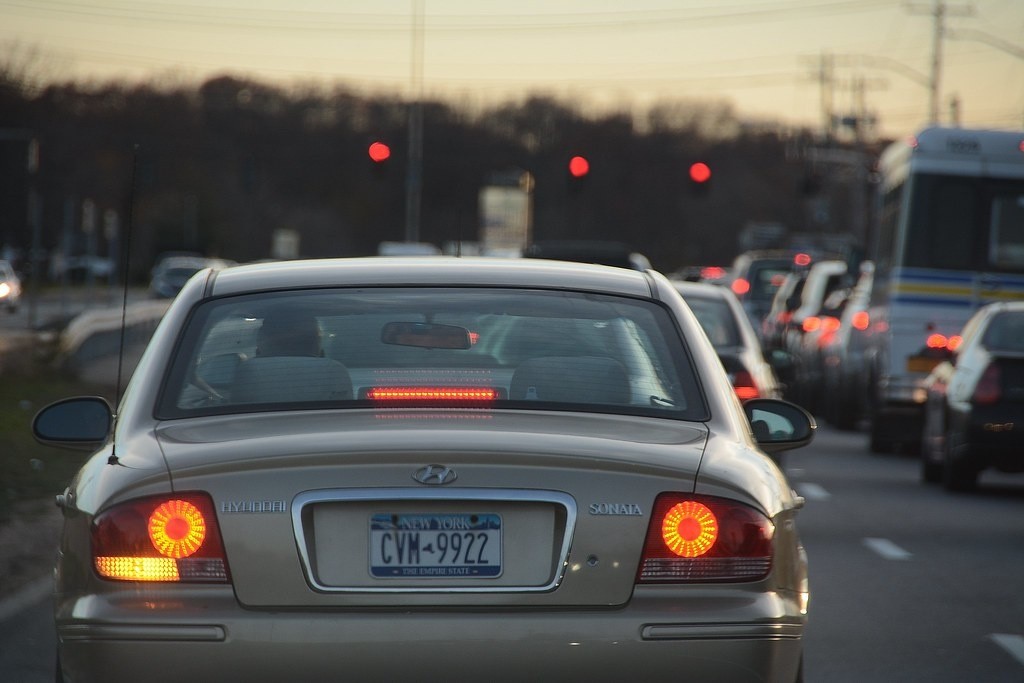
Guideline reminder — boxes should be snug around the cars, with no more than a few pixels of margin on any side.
[28,250,818,683]
[474,275,796,463]
[151,256,223,299]
[0,261,21,313]
[916,300,1024,489]
[672,248,872,425]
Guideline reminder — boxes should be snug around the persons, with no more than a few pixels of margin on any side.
[256,314,325,357]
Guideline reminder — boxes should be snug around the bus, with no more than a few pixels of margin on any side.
[864,125,1024,453]
